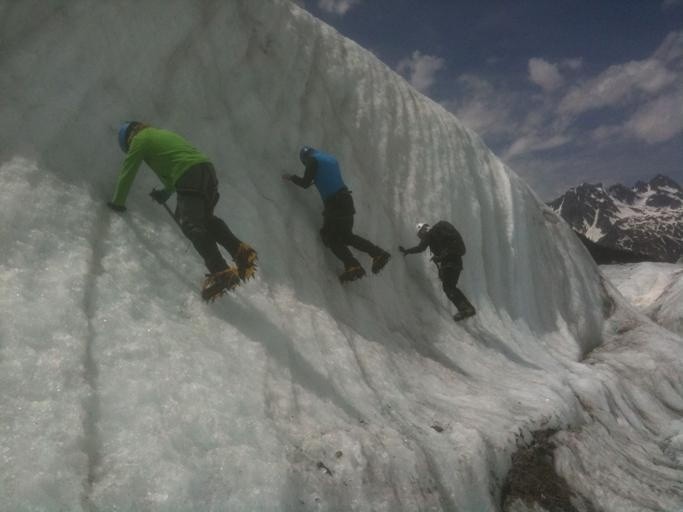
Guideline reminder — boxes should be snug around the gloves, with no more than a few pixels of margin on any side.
[149,188,170,204]
[398,245,409,256]
[107,203,127,213]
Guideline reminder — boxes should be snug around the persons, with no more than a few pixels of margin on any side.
[108,122,257,300]
[398,221,475,321]
[283,146,392,282]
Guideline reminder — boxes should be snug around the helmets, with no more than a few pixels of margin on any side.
[299,145,313,164]
[118,120,138,154]
[415,222,425,235]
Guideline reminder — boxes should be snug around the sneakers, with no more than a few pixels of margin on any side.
[340,264,369,283]
[201,242,259,300]
[453,304,476,321]
[370,250,391,274]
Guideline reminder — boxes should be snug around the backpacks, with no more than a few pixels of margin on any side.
[431,220,466,256]
